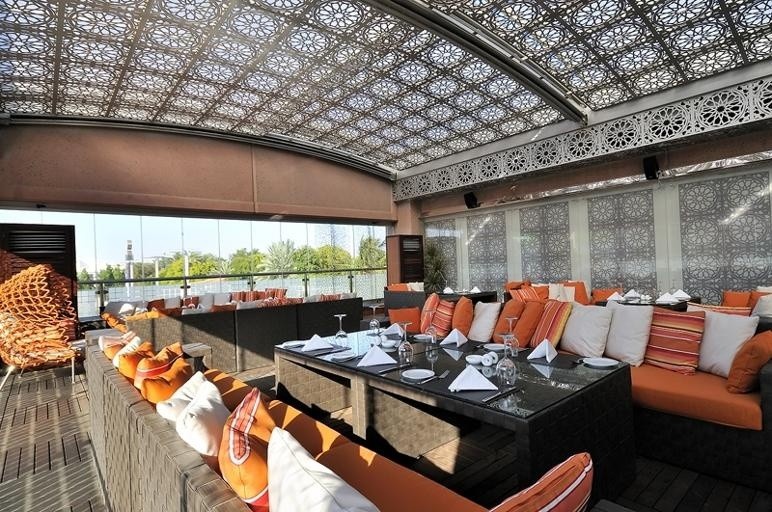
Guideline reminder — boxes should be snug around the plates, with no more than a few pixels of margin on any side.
[483,342,507,352]
[582,357,619,369]
[412,333,432,340]
[402,368,436,380]
[329,351,359,360]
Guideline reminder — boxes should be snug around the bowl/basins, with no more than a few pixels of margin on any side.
[466,364,483,370]
[382,348,396,353]
[381,340,396,348]
[466,354,482,365]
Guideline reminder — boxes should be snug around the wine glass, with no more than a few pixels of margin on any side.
[366,304,382,335]
[503,315,521,358]
[443,279,450,290]
[423,307,438,344]
[425,345,441,371]
[494,331,519,387]
[334,313,350,348]
[180,295,195,310]
[396,322,415,365]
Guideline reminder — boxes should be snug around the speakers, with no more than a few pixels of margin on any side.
[642,155,659,180]
[463,193,478,209]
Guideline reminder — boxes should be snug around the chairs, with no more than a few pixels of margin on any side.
[0,263,79,391]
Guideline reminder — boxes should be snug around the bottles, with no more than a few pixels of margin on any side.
[641,294,651,302]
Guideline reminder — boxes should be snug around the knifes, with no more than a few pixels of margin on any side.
[314,348,345,356]
[378,363,410,375]
[481,386,518,403]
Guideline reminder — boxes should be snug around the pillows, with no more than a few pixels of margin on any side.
[267,426,380,511]
[118,341,157,385]
[511,302,545,347]
[429,298,454,336]
[175,380,232,457]
[725,331,771,395]
[559,300,613,359]
[468,301,501,343]
[686,305,760,380]
[451,296,473,340]
[102,334,135,359]
[420,293,440,334]
[141,357,194,406]
[605,300,655,368]
[528,299,573,349]
[492,299,525,344]
[387,282,771,317]
[217,387,278,511]
[643,305,705,376]
[114,337,142,369]
[100,331,137,351]
[134,340,185,390]
[100,282,357,336]
[155,370,208,422]
[490,452,596,512]
[387,307,421,334]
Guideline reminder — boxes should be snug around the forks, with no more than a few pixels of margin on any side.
[568,358,585,365]
[474,342,484,350]
[417,369,451,386]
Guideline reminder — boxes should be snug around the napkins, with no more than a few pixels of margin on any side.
[439,329,469,348]
[448,365,498,393]
[527,339,559,364]
[301,334,333,353]
[443,348,463,362]
[378,323,405,338]
[356,344,398,368]
[530,364,553,379]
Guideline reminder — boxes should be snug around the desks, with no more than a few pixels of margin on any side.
[275,328,634,511]
